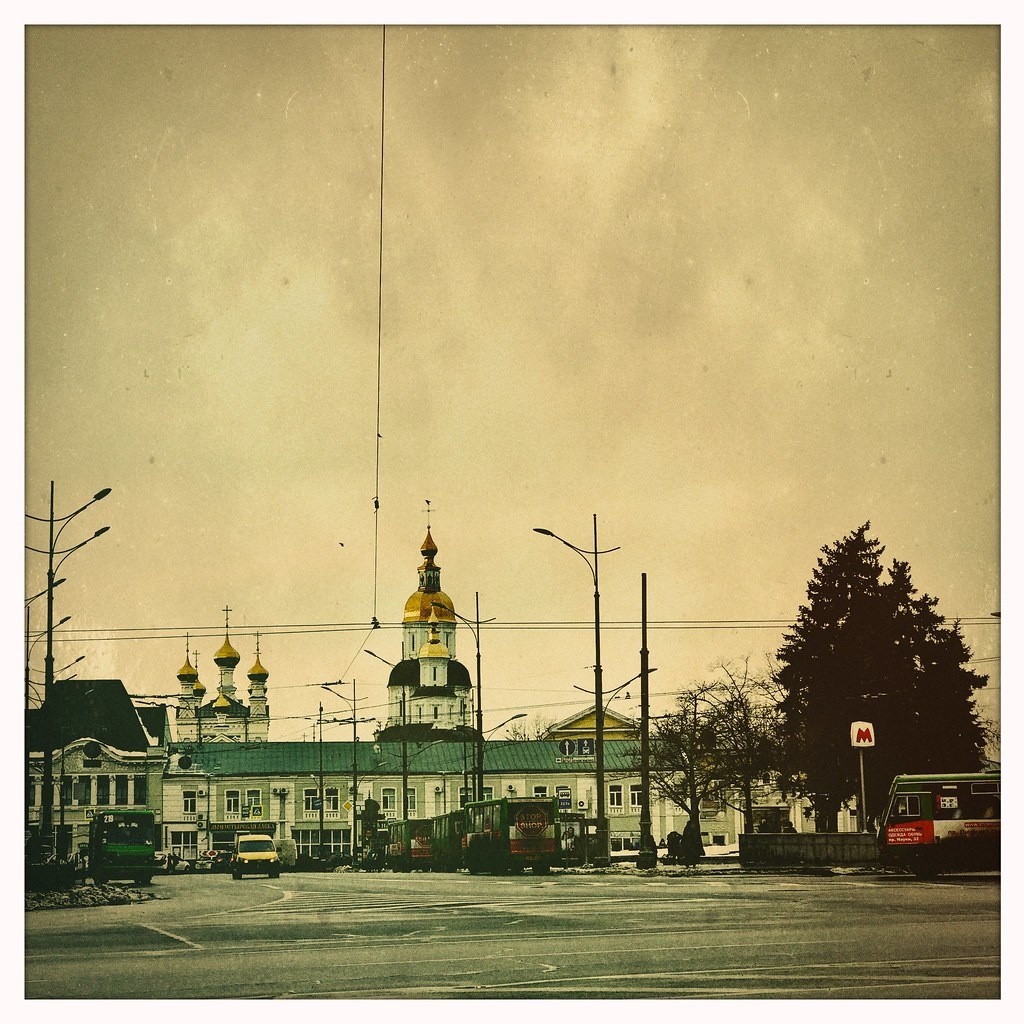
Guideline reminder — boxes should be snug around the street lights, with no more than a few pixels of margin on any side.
[365,641,447,872]
[532,511,658,867]
[429,591,528,798]
[322,678,389,867]
[24,480,113,865]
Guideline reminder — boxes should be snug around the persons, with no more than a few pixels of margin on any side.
[680,820,699,869]
[561,827,579,851]
[759,819,798,833]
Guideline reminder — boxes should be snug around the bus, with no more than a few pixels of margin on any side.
[464,796,562,875]
[874,769,1001,875]
[432,809,469,873]
[89,810,155,885]
[384,818,433,873]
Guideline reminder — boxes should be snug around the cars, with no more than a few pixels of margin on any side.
[195,854,233,872]
[155,853,191,874]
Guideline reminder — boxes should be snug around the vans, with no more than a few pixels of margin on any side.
[231,835,281,880]
